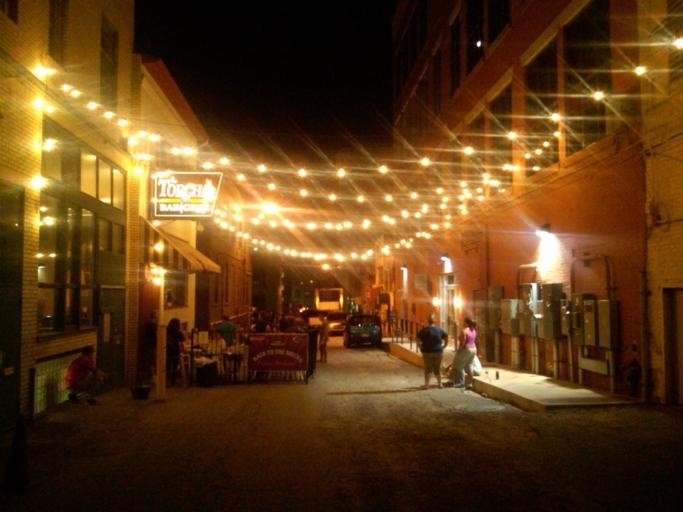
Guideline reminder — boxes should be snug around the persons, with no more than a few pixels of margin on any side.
[451,317,477,390]
[167,318,186,388]
[416,313,449,390]
[63,345,105,405]
[445,352,484,376]
[215,308,330,382]
[137,311,157,388]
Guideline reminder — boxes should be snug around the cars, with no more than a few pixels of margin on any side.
[324,311,383,348]
